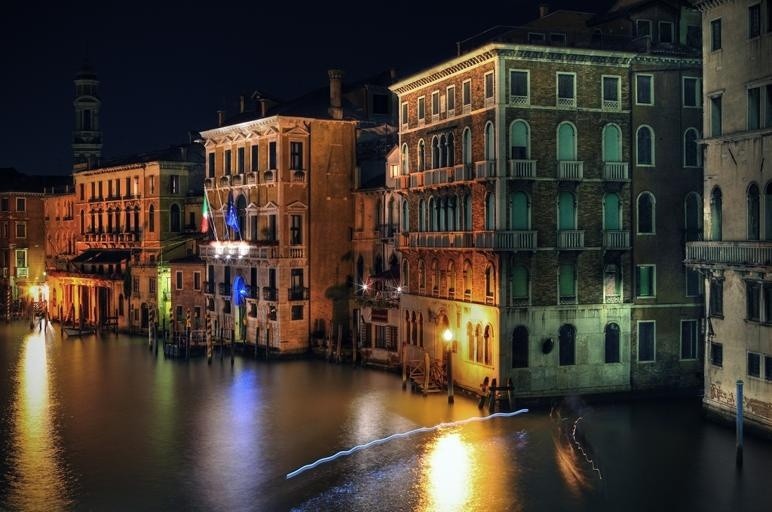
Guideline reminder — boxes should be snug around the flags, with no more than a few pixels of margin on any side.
[198,191,209,233]
[226,191,240,232]
